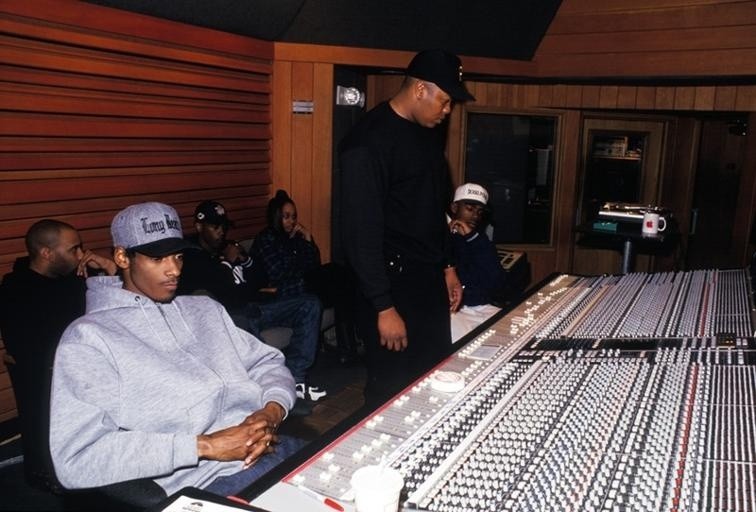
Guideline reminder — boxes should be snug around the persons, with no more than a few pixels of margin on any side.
[48,201,311,500]
[0,218,119,380]
[176,198,329,404]
[248,189,369,368]
[446,180,512,344]
[329,45,480,409]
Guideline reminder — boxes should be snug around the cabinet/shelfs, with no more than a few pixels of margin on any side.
[459,106,669,249]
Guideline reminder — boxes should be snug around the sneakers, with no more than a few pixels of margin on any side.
[294,383,327,402]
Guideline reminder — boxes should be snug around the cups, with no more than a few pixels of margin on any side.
[641,213,667,233]
[351,465,404,512]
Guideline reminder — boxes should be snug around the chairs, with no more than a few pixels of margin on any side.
[0,238,330,512]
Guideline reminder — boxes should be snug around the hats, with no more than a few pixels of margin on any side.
[453,183,493,213]
[111,200,204,259]
[406,48,477,101]
[194,201,235,226]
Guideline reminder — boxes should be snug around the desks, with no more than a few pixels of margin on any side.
[236,270,557,500]
